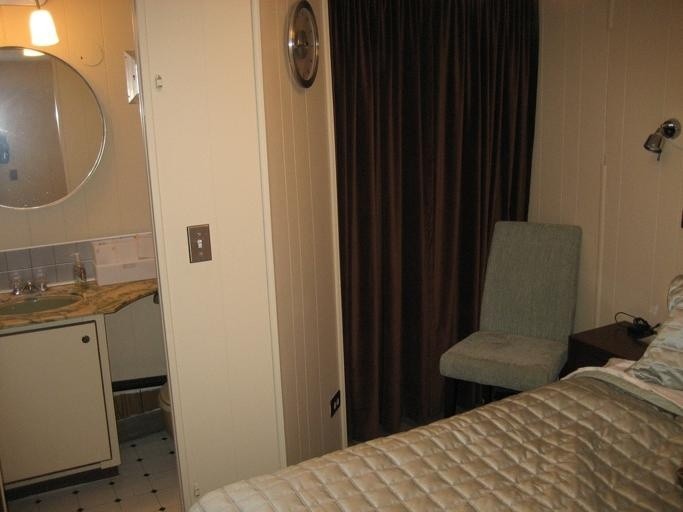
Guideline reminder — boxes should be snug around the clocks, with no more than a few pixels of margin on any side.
[282,0,320,89]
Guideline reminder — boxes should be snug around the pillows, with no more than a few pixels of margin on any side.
[623,272,683,391]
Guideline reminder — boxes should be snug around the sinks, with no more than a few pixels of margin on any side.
[0,292,82,316]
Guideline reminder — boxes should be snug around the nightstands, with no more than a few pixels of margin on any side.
[567,321,658,368]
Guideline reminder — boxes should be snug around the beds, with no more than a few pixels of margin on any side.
[175,357,681,512]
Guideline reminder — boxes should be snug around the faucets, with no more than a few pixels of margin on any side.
[24,281,38,294]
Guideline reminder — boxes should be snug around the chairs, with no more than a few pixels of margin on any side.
[438,221,582,419]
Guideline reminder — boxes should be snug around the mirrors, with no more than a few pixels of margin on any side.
[0,45,107,213]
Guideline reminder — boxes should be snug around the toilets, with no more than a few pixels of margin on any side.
[158,381,172,430]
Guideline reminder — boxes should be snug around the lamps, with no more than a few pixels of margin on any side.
[642,117,681,162]
[30,0,60,48]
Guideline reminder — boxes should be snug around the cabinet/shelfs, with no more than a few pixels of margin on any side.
[1,314,122,503]
